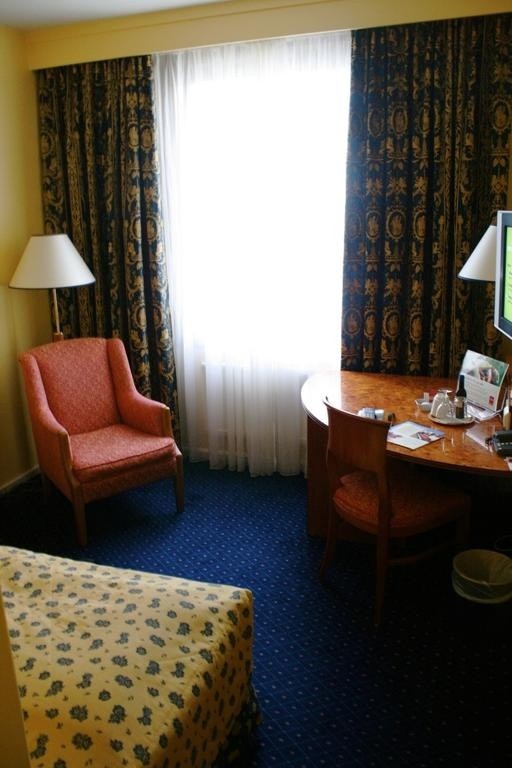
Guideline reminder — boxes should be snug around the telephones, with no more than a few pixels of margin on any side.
[492,430,512,455]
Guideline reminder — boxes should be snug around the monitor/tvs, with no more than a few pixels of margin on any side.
[494,210,512,341]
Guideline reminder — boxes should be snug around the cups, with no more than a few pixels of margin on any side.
[430,393,442,416]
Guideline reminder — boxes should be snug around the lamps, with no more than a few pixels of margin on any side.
[456,225,496,282]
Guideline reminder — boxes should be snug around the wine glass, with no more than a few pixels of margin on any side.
[437,388,453,418]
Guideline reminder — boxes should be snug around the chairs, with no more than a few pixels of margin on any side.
[20,338,183,544]
[10,234,97,341]
[320,396,472,629]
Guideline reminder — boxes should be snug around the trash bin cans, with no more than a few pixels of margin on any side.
[452,549,512,617]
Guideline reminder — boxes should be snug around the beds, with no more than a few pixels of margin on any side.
[1,543,254,765]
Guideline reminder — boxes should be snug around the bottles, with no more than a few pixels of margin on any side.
[455,375,466,418]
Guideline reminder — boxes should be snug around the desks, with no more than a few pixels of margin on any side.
[300,365,511,538]
